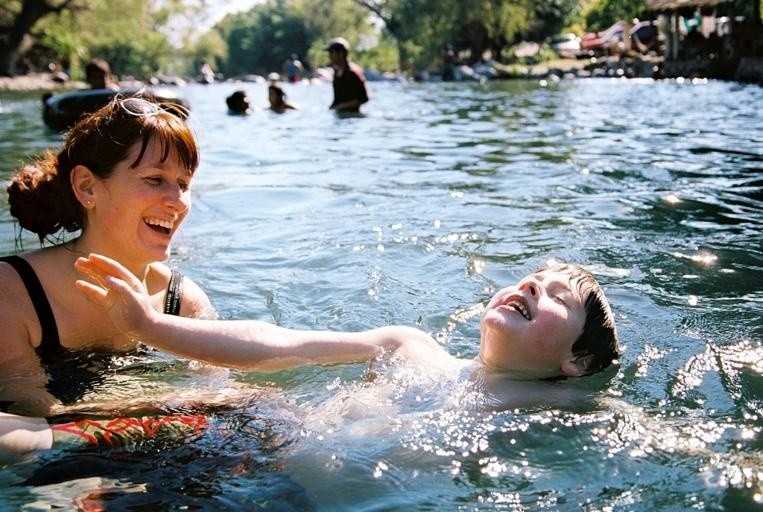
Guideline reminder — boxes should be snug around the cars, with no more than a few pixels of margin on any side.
[544,18,665,58]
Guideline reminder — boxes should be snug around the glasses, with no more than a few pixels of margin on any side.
[117,96,160,121]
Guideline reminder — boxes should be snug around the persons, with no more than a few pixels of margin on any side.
[197,57,215,84]
[268,86,295,114]
[225,91,250,114]
[589,10,761,61]
[322,37,370,113]
[84,59,118,90]
[284,53,305,82]
[0,91,310,512]
[268,73,280,86]
[0,253,712,512]
